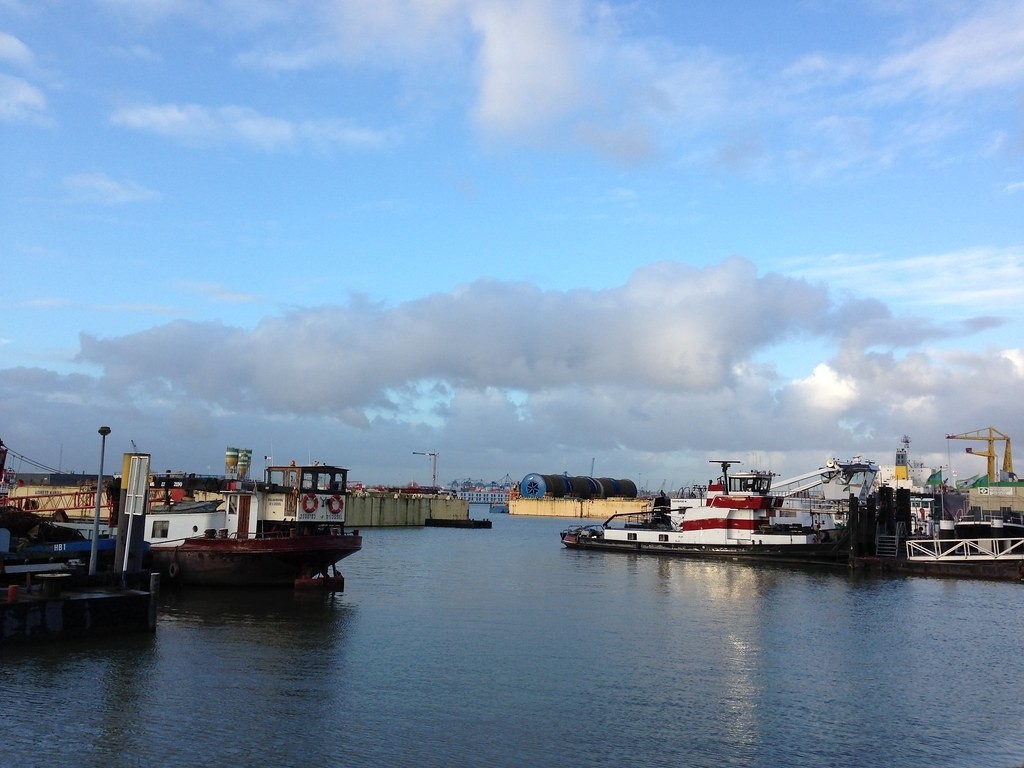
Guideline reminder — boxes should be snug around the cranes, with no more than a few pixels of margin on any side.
[412,447,441,490]
[944,427,1013,485]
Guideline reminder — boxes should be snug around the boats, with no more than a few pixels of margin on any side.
[0,437,365,603]
[558,446,1024,584]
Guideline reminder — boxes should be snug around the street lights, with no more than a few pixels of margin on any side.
[86,425,113,591]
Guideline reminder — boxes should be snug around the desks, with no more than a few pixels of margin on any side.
[35,573,72,598]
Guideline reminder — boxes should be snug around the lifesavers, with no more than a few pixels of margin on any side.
[169,563,179,578]
[303,493,318,513]
[814,536,821,544]
[329,494,343,514]
